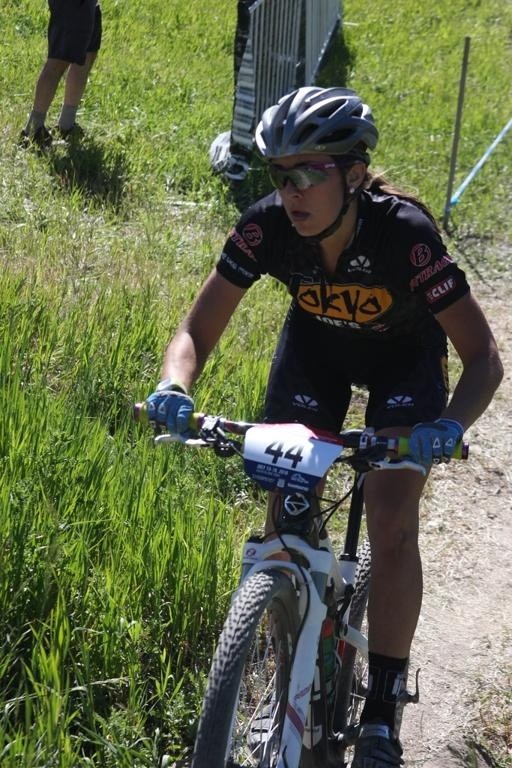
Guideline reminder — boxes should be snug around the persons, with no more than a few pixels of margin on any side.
[18,0,103,157]
[139,84,505,766]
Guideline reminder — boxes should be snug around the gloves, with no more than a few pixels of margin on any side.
[407,414,466,467]
[141,391,196,438]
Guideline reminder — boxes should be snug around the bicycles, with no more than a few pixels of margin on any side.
[133,400,470,768]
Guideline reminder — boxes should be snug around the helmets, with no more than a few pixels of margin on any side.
[249,84,380,167]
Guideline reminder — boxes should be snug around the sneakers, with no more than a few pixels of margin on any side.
[52,121,87,142]
[350,722,405,768]
[16,127,54,152]
[246,702,281,760]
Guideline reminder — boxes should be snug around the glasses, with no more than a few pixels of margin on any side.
[267,160,364,193]
